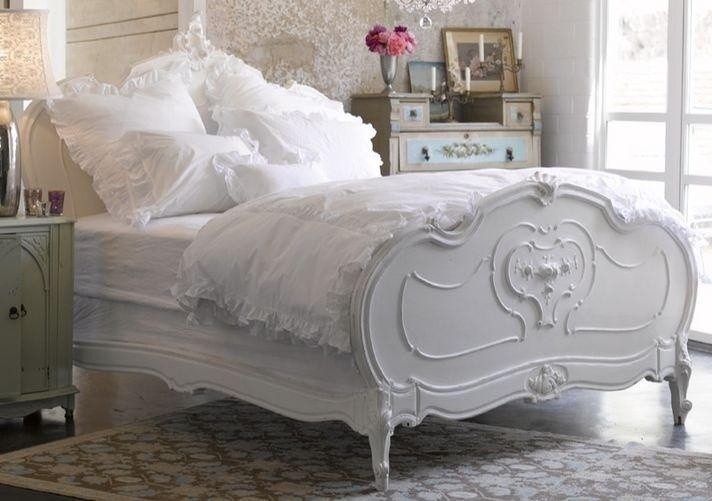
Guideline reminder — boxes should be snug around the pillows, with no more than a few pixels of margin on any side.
[44,77,206,229]
[212,105,383,181]
[113,126,260,232]
[208,70,344,110]
[213,150,326,207]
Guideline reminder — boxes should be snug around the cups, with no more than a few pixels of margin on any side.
[47,188,65,215]
[23,186,43,217]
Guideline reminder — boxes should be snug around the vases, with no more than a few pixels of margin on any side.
[380,54,397,95]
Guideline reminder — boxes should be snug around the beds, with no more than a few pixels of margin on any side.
[19,20,700,493]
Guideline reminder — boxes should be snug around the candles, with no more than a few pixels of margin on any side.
[479,34,484,62]
[517,31,523,59]
[431,66,436,90]
[465,67,471,91]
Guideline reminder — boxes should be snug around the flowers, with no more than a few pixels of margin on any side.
[365,23,415,56]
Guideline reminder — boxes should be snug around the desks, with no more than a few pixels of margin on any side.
[350,92,542,175]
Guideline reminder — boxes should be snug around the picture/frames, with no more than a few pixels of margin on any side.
[441,27,519,92]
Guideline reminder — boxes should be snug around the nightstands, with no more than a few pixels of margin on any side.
[0,216,80,437]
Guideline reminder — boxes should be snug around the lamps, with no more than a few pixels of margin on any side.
[0,8,64,216]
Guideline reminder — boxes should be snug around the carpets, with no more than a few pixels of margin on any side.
[0,397,712,501]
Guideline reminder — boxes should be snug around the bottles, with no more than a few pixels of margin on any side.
[1,101,24,218]
